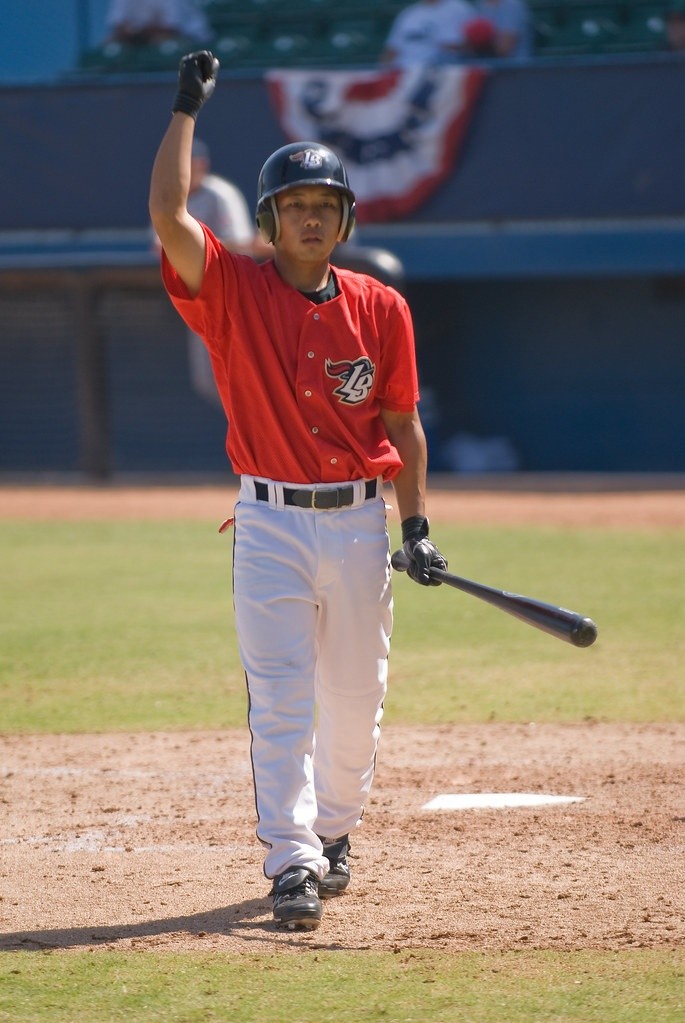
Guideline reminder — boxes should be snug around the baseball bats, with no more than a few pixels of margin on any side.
[391,549,599,648]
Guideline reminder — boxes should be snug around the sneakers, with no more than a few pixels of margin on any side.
[268,865,321,931]
[316,833,351,896]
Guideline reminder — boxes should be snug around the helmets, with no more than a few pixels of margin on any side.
[255,142,357,244]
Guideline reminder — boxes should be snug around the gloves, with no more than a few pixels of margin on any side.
[401,515,448,586]
[171,50,220,122]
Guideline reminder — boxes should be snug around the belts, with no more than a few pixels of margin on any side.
[254,477,377,511]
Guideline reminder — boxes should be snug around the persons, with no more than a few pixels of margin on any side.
[106,0,208,50]
[668,0,685,48]
[382,0,530,60]
[154,143,276,398]
[149,50,449,928]
